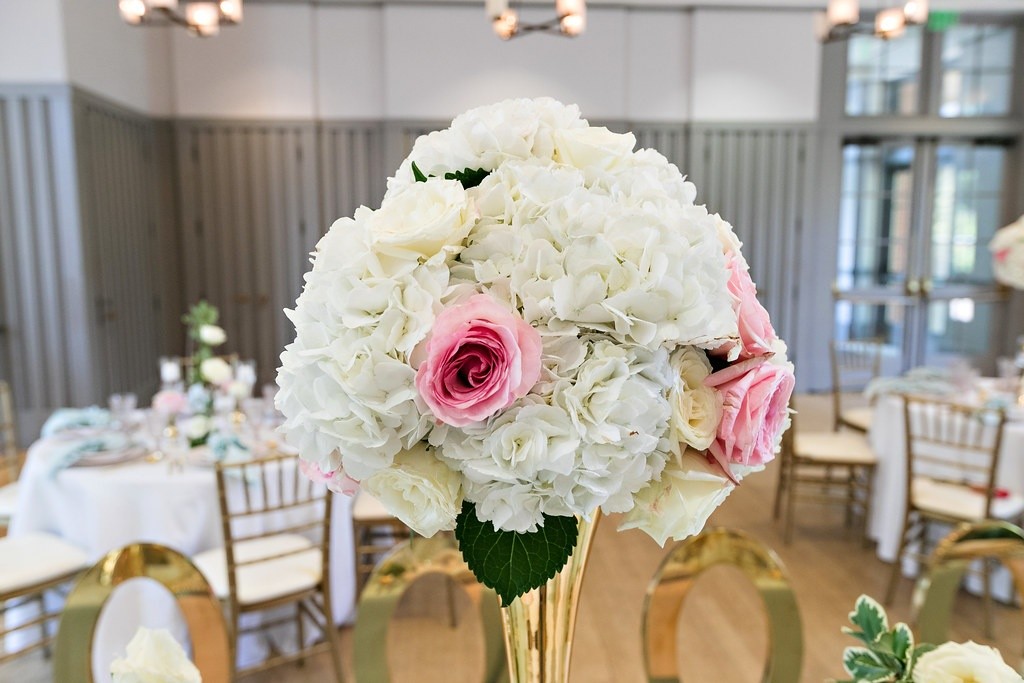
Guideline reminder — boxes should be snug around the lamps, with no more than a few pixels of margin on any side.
[120,0,247,36]
[486,0,588,40]
[813,0,928,40]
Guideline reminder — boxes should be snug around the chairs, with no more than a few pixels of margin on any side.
[820,333,884,496]
[769,391,876,541]
[882,394,1007,637]
[1,354,454,683]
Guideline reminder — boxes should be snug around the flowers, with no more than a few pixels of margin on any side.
[152,309,262,452]
[275,97,796,603]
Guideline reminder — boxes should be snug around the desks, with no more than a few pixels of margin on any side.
[15,420,362,665]
[871,398,1024,605]
